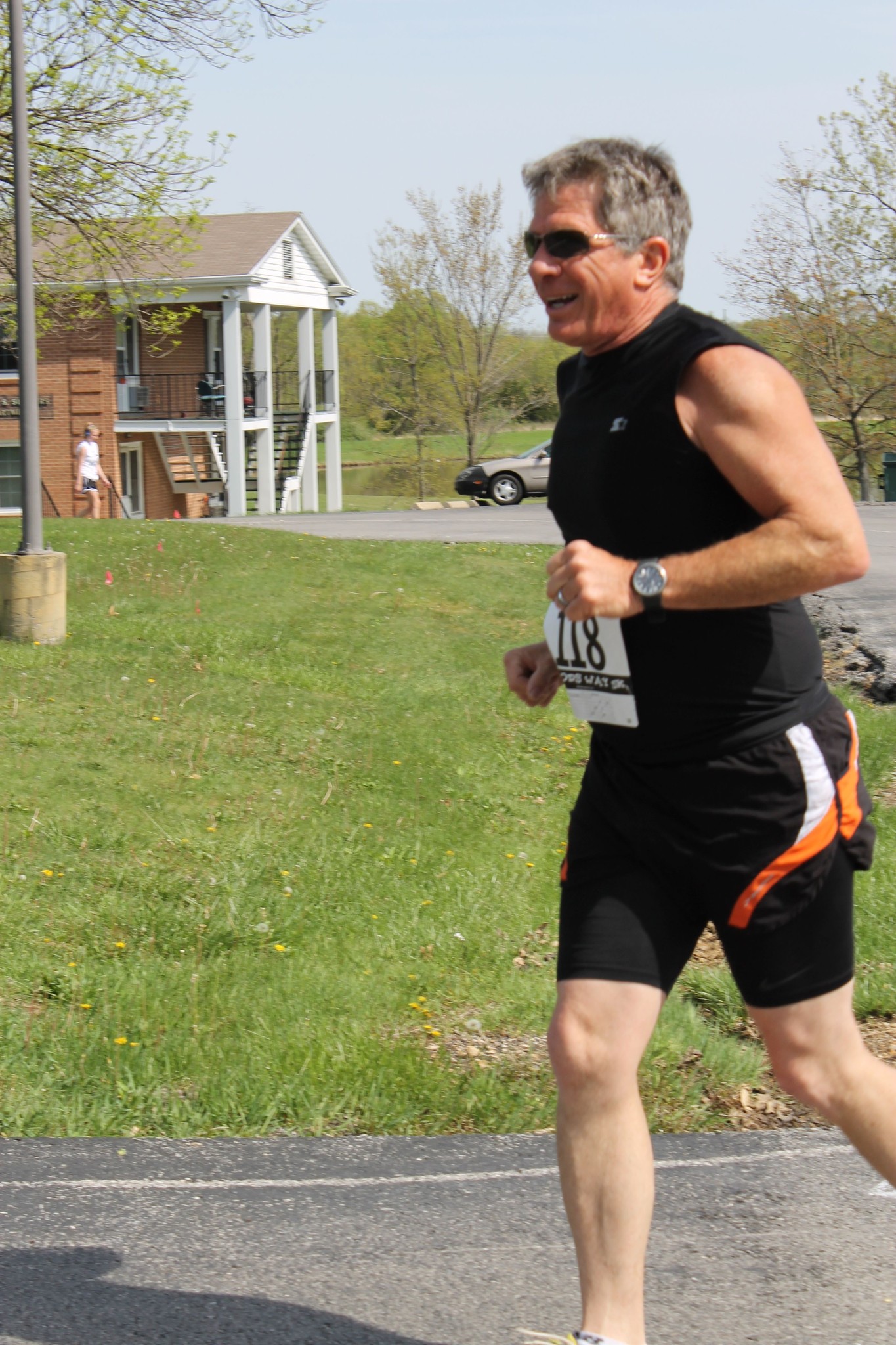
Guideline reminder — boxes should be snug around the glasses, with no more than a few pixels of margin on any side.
[524,227,649,262]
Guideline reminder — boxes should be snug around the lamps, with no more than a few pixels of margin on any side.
[335,299,344,305]
[220,287,243,300]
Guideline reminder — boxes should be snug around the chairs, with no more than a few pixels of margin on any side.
[195,380,226,418]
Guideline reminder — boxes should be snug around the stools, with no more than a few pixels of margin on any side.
[244,397,255,417]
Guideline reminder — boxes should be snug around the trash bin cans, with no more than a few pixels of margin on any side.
[877,452,896,501]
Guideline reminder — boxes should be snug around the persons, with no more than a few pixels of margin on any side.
[72,422,112,519]
[503,138,896,1345]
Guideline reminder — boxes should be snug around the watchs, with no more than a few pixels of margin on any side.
[632,556,668,614]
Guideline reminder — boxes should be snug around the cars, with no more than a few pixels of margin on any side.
[455,439,552,506]
